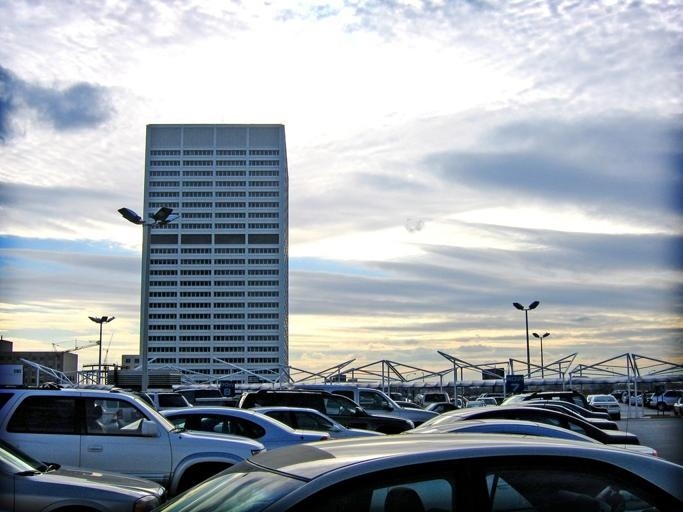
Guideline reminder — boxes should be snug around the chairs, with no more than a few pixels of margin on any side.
[387,488,425,511]
[91,405,108,432]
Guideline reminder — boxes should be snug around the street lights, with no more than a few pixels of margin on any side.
[532,332,550,378]
[512,300,539,378]
[118,205,172,392]
[87,315,114,386]
